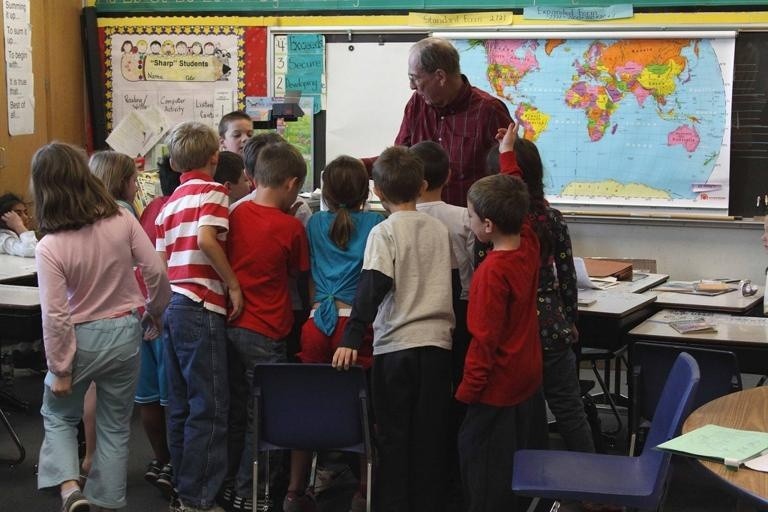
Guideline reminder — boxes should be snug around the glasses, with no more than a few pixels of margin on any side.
[407,71,438,81]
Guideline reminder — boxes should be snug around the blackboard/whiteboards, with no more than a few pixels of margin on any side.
[266,27,768,225]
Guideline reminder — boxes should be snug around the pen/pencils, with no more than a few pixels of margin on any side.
[118,52,233,83]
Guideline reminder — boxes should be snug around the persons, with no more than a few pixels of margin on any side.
[761,215,768,317]
[0,192,38,258]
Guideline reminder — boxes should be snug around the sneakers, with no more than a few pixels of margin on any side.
[351,491,367,512]
[62,489,90,512]
[282,490,321,512]
[144,460,165,492]
[167,487,276,512]
[156,463,175,490]
[314,463,352,494]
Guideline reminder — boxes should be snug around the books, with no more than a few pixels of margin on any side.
[669,317,718,335]
[650,275,741,297]
[649,423,768,472]
[584,253,633,279]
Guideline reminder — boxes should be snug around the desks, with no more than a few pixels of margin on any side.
[566,262,768,390]
[682,385,768,512]
[0,250,42,343]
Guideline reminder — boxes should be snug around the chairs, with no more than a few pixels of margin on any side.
[248,362,375,512]
[622,339,768,457]
[0,409,26,469]
[548,257,657,436]
[509,350,703,512]
